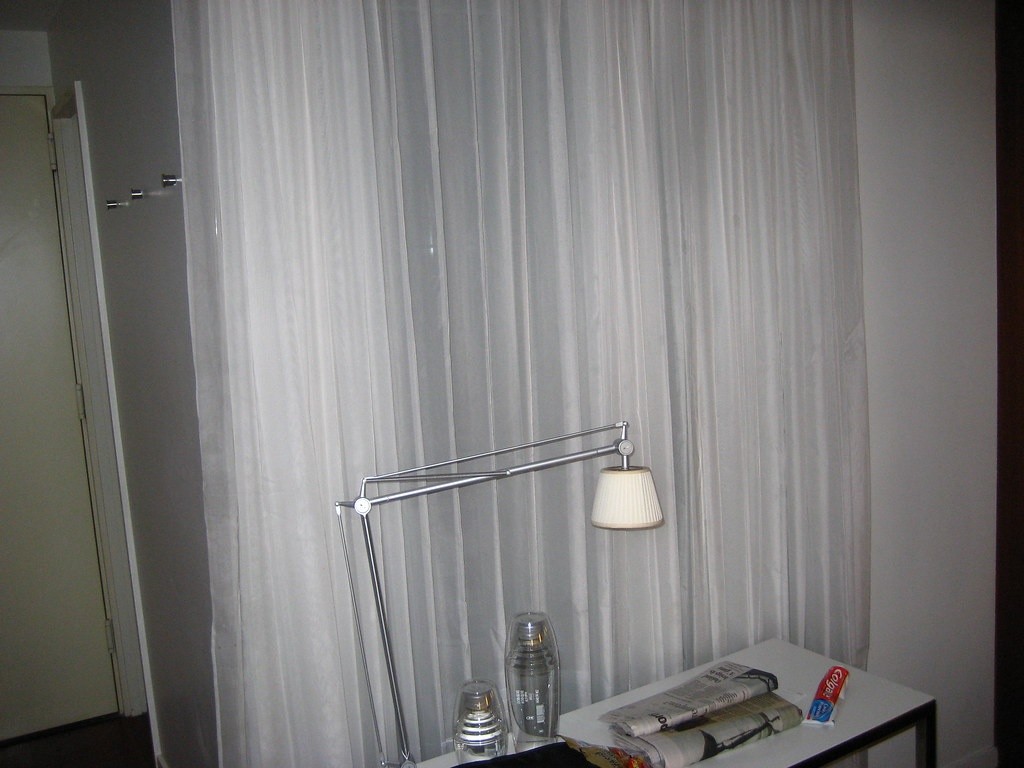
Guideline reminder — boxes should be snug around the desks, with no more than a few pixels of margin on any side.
[416,636,937,768]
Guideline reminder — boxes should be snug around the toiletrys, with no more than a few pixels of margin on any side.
[800,664,850,727]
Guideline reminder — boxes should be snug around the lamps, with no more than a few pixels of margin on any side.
[335,422,665,768]
